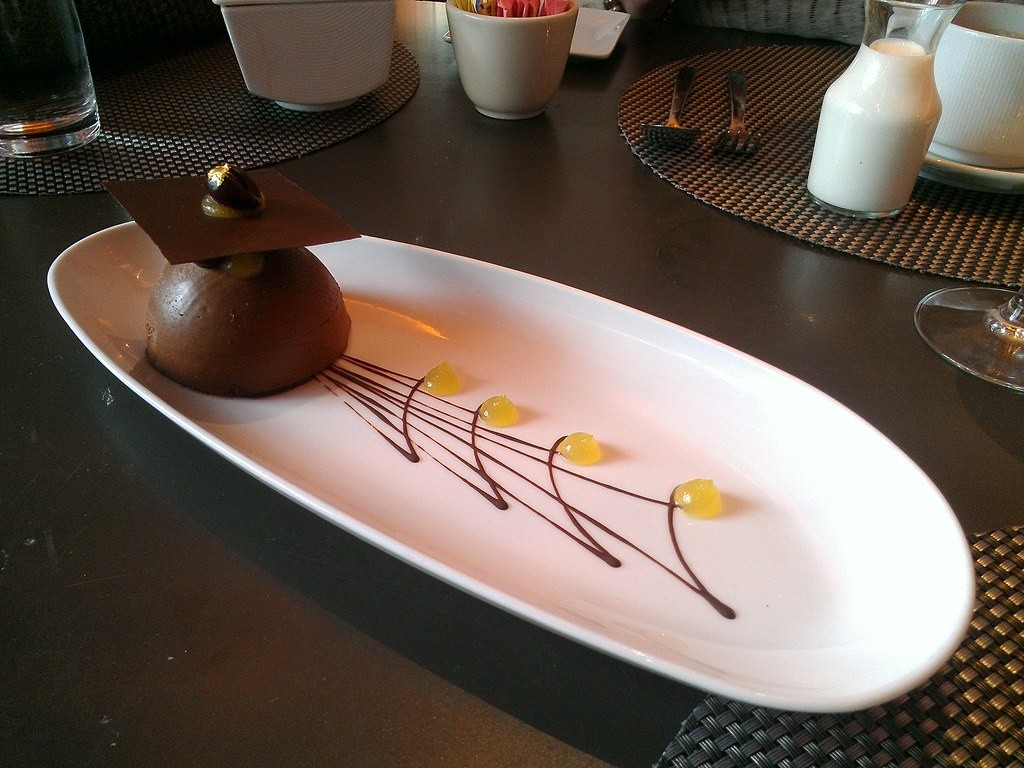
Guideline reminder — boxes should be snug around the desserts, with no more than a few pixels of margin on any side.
[675,479,721,518]
[424,362,459,397]
[559,432,601,465]
[479,396,517,426]
[146,248,351,395]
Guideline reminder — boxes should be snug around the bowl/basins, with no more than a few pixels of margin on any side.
[925,2,1024,169]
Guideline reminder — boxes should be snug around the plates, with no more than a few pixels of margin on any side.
[919,153,1024,195]
[47,213,976,712]
[443,7,631,58]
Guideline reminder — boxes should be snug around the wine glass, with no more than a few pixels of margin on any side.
[913,284,1024,392]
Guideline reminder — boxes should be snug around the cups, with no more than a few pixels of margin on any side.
[446,0,581,120]
[0,0,101,159]
[212,0,397,112]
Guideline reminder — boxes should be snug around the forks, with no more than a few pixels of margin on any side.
[713,69,764,163]
[638,64,701,154]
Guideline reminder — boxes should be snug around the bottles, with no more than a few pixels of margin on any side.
[805,1,966,222]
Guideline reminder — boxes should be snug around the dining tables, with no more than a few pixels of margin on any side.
[2,1,1023,768]
